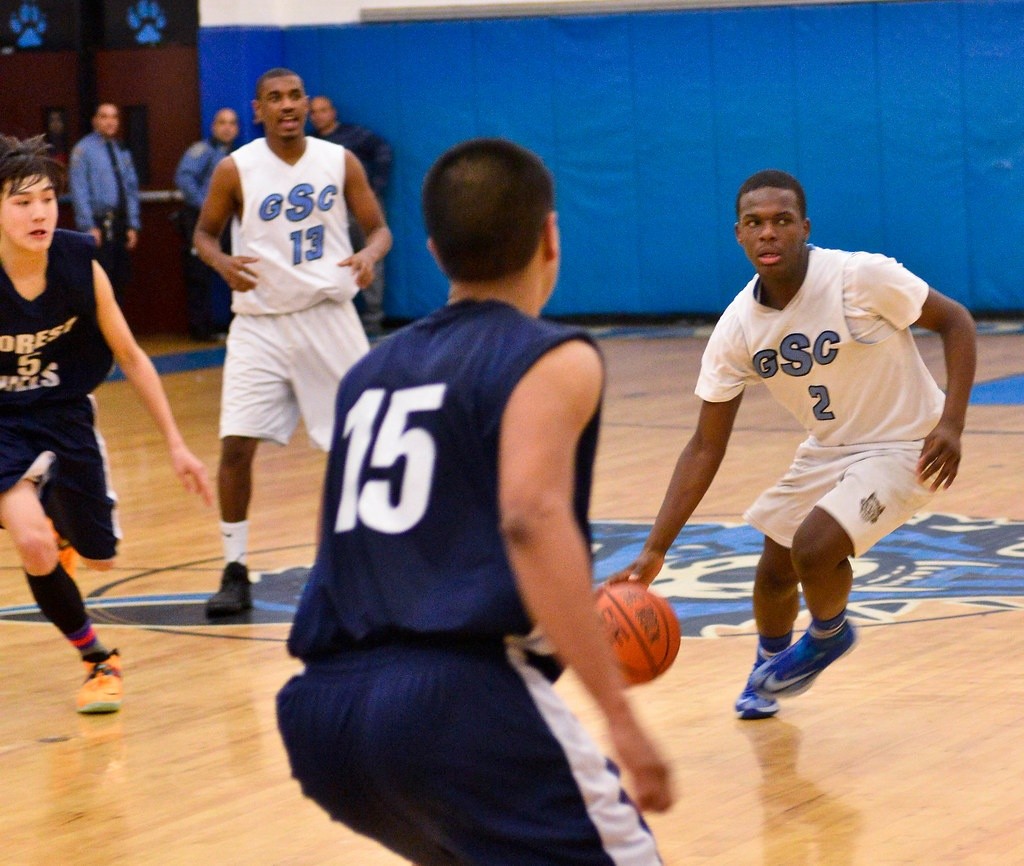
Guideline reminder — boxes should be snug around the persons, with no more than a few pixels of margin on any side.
[304,96,396,345]
[192,66,392,616]
[1,135,214,716]
[177,109,239,343]
[69,102,142,302]
[276,139,676,866]
[605,169,979,719]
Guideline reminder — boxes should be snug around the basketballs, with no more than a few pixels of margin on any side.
[590,585,684,686]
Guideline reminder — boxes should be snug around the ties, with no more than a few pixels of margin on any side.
[105,142,129,228]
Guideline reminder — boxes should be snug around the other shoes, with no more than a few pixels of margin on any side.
[364,324,383,340]
[191,330,220,344]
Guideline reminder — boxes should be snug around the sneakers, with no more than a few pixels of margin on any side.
[74,649,125,713]
[205,560,253,616]
[47,513,76,578]
[734,654,782,720]
[751,619,858,698]
